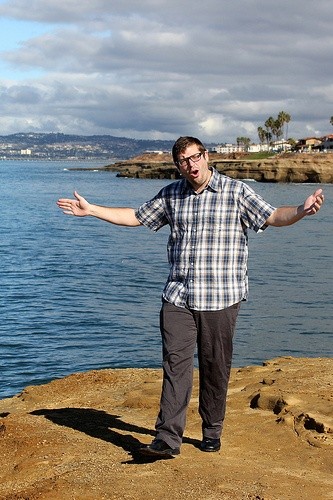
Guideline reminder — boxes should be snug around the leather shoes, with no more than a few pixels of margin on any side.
[139,438,180,456]
[200,434,221,452]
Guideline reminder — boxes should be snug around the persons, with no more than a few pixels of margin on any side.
[55,136,325,455]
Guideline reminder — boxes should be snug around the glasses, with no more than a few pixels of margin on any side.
[175,151,204,166]
